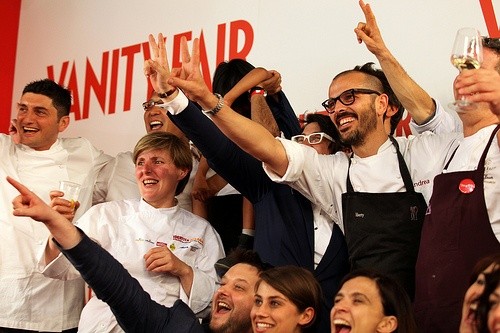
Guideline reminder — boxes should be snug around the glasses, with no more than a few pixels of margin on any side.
[143,100,165,110]
[291,132,336,144]
[322,88,381,113]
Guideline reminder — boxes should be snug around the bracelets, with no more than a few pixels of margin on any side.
[155,88,178,98]
[201,92,224,117]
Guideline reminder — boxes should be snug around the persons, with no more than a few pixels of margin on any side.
[0,78,118,333]
[35,130,226,333]
[6,177,274,333]
[141,0,500,333]
[91,87,200,221]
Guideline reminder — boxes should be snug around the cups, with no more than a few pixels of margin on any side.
[59,181,82,222]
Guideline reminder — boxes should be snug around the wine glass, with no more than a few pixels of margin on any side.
[448,29,483,112]
